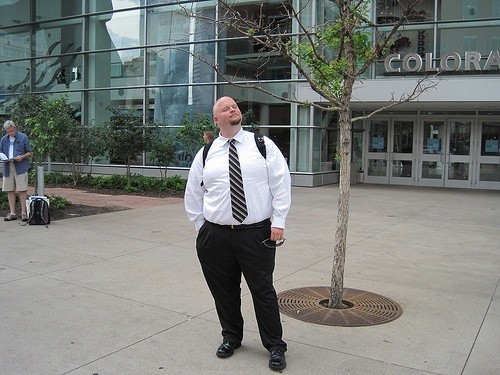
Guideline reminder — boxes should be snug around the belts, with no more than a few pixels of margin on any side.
[218,218,270,230]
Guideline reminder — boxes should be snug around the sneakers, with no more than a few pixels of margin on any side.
[4,213,18,221]
[21,214,29,221]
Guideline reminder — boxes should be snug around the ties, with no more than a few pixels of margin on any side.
[227,138,249,223]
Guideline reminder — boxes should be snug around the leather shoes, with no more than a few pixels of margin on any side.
[216,340,242,358]
[269,349,287,370]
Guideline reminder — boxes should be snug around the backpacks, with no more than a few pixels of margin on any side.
[21,197,51,228]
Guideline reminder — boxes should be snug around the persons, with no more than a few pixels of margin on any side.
[0,120,32,222]
[184,96,291,371]
